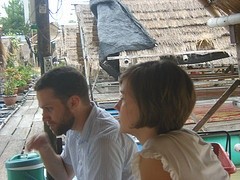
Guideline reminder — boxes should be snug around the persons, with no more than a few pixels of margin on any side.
[114,60,230,180]
[25,66,141,180]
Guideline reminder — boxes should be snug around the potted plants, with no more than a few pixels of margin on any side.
[2,65,32,106]
